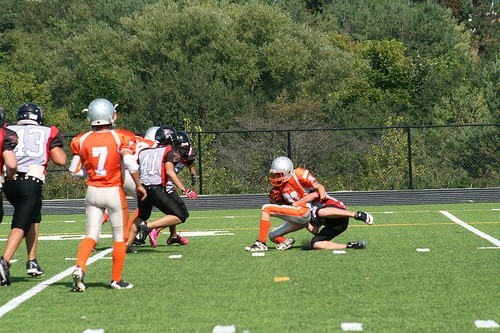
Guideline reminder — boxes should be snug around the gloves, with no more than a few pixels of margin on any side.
[182,187,197,200]
[191,174,198,185]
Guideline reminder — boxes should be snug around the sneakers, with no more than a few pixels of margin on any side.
[27,258,44,277]
[138,221,151,244]
[346,241,366,249]
[0,259,11,285]
[72,267,86,292]
[167,235,189,245]
[126,248,137,253]
[245,240,268,251]
[111,279,133,289]
[276,237,295,250]
[134,235,140,245]
[148,228,158,247]
[355,211,373,225]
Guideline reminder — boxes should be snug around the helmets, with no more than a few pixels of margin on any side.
[0,107,6,127]
[175,132,190,149]
[86,99,113,126]
[268,157,293,186]
[162,127,176,145]
[17,103,40,124]
[145,126,167,144]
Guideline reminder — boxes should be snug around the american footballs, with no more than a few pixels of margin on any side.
[269,188,286,205]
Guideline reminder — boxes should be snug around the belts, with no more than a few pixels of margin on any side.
[13,176,42,184]
[144,185,162,189]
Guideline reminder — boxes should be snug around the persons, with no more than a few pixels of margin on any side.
[245,157,374,251]
[0,99,201,292]
